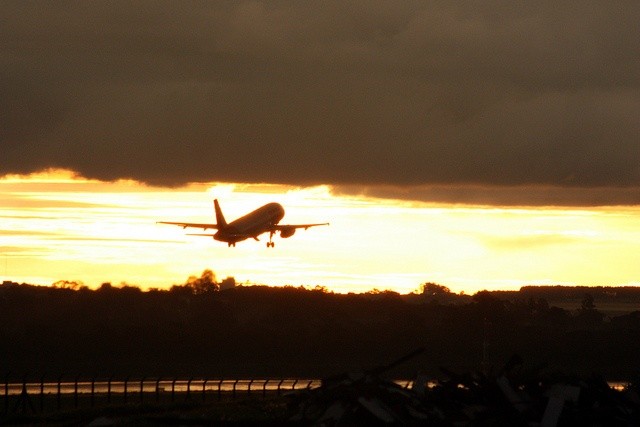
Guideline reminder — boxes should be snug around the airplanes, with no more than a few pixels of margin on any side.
[158,199,329,247]
